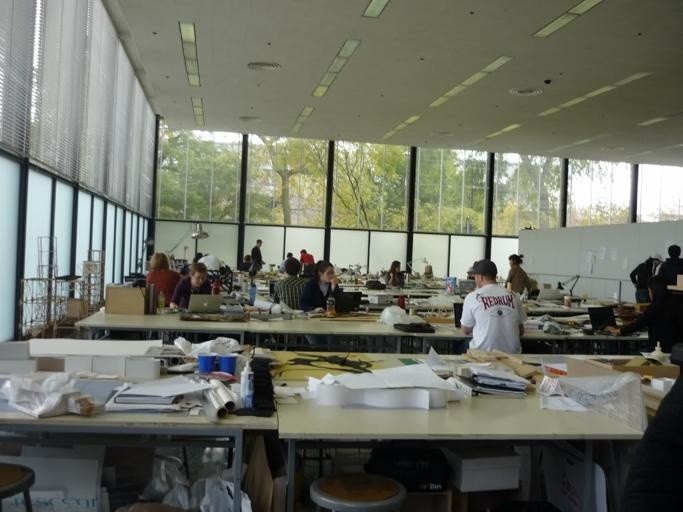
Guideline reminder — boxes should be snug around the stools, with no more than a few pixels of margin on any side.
[1,462,36,511]
[310,474,407,511]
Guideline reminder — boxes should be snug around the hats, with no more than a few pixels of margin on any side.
[650,253,665,264]
[467,259,497,275]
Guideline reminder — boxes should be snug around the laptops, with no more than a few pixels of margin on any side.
[587,307,640,337]
[453,302,475,328]
[184,294,222,313]
[334,292,362,312]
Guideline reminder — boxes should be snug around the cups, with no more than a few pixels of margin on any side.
[218,354,238,375]
[197,352,218,373]
[563,296,571,307]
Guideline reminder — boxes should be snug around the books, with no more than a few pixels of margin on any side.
[452,362,529,398]
[104,374,215,412]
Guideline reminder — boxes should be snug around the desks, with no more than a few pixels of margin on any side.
[0,267,682,354]
[1,342,278,512]
[273,351,645,510]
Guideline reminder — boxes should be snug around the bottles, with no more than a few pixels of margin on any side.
[158,290,166,310]
[325,296,335,317]
[398,295,405,309]
[148,283,156,314]
[212,277,221,296]
[649,342,665,363]
[249,284,257,302]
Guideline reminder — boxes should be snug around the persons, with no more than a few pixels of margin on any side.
[147,240,350,313]
[629,252,664,303]
[619,372,683,512]
[605,275,682,353]
[384,261,404,288]
[459,259,529,355]
[504,254,532,297]
[658,245,683,285]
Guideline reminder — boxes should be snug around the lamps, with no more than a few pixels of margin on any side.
[189,220,210,262]
